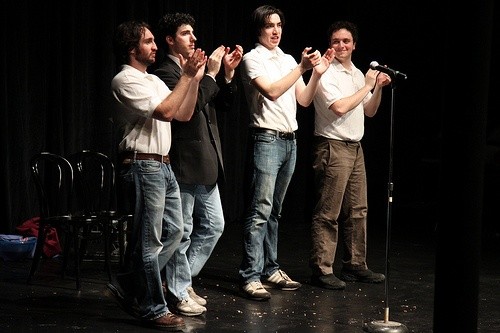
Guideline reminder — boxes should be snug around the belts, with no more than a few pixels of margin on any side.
[120,151,170,165]
[251,125,298,140]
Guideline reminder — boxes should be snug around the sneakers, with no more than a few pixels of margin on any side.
[236,277,271,300]
[344,262,385,283]
[142,308,185,330]
[303,263,345,289]
[186,287,207,306]
[260,268,302,290]
[106,280,148,318]
[169,295,207,316]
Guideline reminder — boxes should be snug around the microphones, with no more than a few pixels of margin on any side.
[370,61,406,80]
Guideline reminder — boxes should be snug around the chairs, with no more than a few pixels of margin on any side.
[64,149,129,266]
[28,147,110,291]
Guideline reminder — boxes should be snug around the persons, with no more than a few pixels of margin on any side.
[108,21,209,329]
[239,6,335,301]
[151,12,244,317]
[305,20,391,290]
